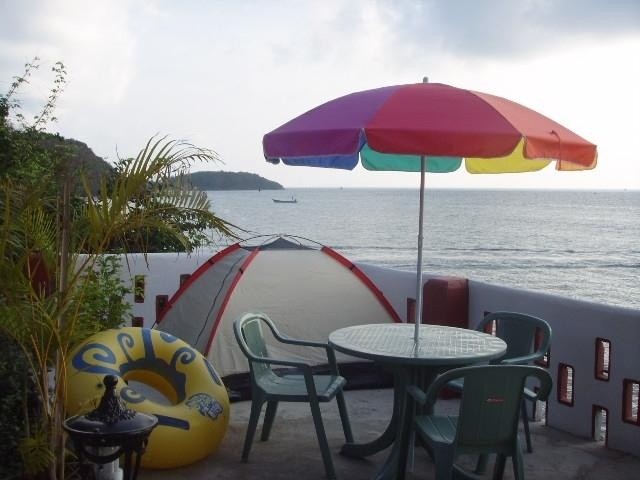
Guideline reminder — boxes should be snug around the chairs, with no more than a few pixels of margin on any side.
[234,311,355,480]
[402,310,554,480]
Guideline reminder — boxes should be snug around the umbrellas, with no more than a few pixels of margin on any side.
[263,77,597,341]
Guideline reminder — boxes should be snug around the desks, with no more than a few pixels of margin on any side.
[327,322,509,478]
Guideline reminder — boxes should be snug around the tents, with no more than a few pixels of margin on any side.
[149,233,404,404]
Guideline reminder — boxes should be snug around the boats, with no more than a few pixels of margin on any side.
[272,199,297,203]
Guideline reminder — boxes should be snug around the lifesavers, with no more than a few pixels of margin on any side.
[58,326,229,469]
